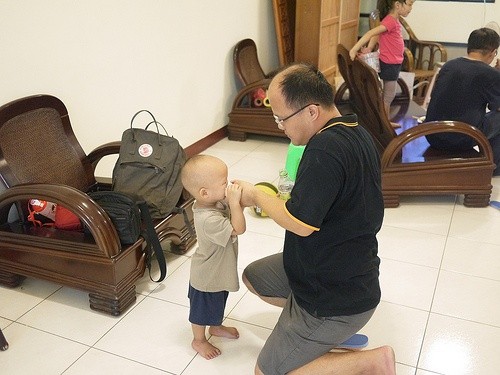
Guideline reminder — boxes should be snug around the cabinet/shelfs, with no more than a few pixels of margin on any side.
[294,0,361,80]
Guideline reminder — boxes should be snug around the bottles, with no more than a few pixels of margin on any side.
[278,170,294,195]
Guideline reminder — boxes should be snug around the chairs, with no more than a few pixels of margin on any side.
[227,38,290,142]
[0,93,197,317]
[333,11,496,209]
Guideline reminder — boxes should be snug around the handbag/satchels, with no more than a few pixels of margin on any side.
[357,50,382,74]
[111,109,185,218]
[84,193,168,282]
[27,200,83,230]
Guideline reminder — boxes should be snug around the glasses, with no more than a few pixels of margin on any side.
[495,50,497,56]
[273,103,320,125]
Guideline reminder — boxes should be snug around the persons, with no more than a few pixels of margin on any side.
[180,154,246,360]
[230,62,395,375]
[349,0,415,128]
[424,29,500,177]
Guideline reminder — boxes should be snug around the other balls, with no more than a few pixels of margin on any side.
[253,182,279,217]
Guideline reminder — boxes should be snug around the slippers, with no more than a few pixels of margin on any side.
[338,335,369,348]
